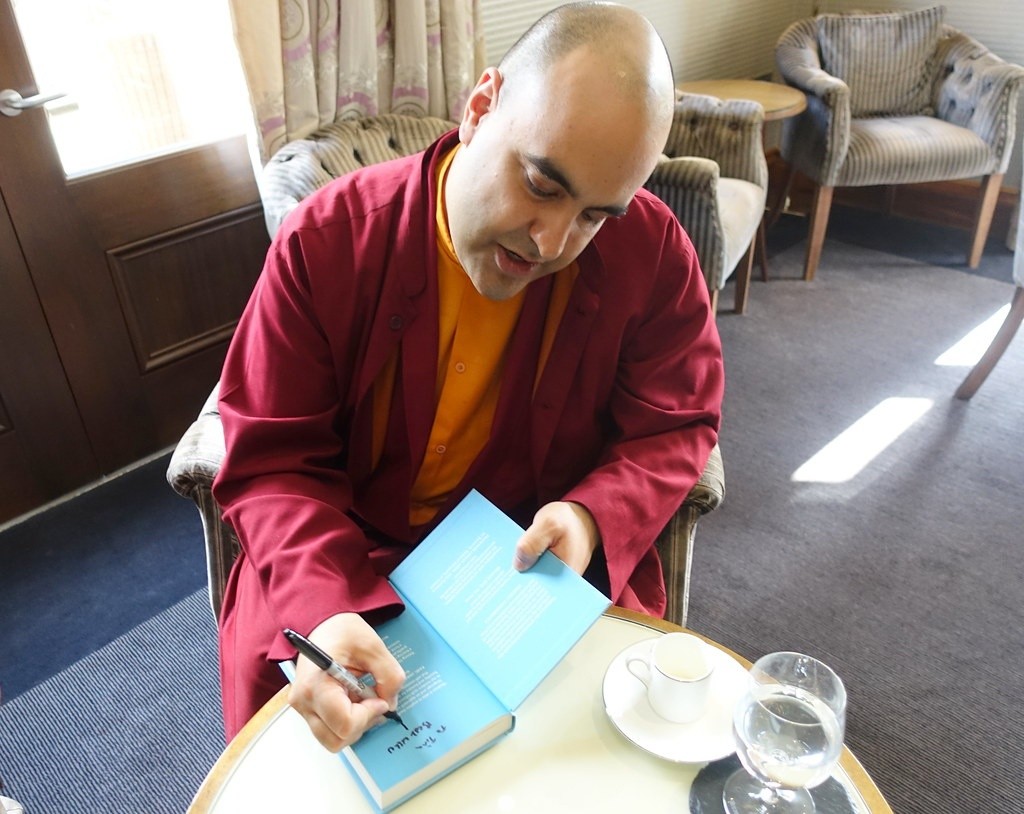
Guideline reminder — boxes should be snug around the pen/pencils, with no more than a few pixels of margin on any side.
[283,626,406,725]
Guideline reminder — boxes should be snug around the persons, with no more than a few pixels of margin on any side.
[218,2,724,750]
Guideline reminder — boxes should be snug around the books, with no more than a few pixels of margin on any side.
[277,488,612,814]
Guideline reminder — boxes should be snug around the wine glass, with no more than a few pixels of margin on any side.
[720,651,849,814]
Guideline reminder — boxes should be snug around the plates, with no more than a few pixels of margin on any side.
[601,636,750,764]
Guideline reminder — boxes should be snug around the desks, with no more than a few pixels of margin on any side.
[181,601,897,814]
[674,77,811,284]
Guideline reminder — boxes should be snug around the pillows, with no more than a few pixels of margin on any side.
[812,4,946,117]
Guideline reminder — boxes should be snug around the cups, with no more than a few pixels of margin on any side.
[626,632,716,726]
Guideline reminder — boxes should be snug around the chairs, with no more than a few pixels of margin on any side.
[258,113,460,243]
[642,88,770,321]
[163,384,722,630]
[772,4,1024,285]
